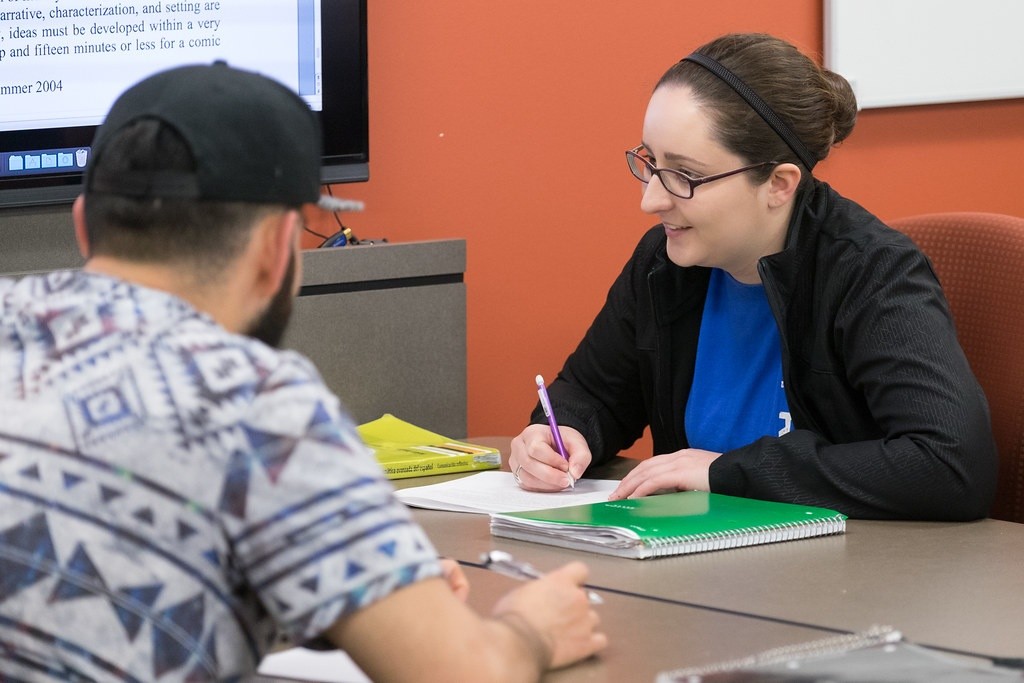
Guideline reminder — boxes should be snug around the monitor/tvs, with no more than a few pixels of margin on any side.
[0,0,370,205]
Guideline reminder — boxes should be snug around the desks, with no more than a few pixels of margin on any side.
[251,436,1024,683]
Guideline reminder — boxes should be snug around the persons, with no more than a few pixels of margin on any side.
[0,57,608,683]
[509,32,998,523]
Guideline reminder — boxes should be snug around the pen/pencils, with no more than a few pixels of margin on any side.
[534,374,573,489]
[476,550,605,609]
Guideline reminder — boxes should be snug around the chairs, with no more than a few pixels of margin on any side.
[881,211,1024,526]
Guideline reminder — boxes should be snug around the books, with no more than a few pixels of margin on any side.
[489,489,849,560]
[354,412,502,480]
[656,622,1023,683]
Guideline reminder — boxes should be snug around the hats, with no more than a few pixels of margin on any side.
[81,58,362,212]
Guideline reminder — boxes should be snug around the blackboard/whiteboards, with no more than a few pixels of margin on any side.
[822,1,1023,108]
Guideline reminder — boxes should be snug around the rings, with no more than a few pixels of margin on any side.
[514,465,522,486]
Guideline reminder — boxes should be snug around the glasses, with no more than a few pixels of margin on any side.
[624,147,784,200]
